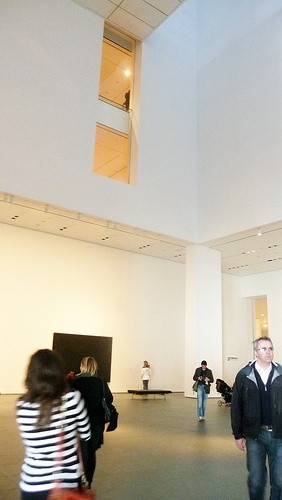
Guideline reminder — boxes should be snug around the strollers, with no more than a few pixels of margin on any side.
[216,379,233,406]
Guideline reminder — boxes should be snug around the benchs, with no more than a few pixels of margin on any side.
[128,389,173,400]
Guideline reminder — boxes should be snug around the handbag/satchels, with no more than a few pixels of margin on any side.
[192,381,198,392]
[48,483,96,500]
[102,398,110,423]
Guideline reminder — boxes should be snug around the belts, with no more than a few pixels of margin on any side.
[261,425,273,431]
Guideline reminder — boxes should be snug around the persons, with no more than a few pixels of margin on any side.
[15,349,91,500]
[68,356,114,491]
[230,335,282,500]
[141,360,152,396]
[193,360,214,422]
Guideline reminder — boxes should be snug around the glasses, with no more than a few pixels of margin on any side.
[257,347,273,352]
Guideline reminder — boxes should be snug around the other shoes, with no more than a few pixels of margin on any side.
[199,416,205,421]
